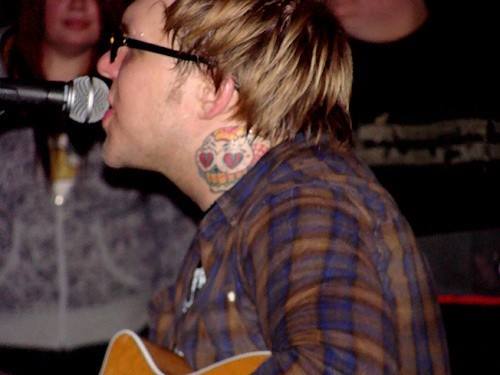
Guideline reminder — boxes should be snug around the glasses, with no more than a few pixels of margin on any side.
[109,27,220,68]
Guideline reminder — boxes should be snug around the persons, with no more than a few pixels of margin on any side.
[93,0,450,375]
[294,0,500,375]
[0,0,206,375]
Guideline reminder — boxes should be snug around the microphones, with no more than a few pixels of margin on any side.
[0,74,111,125]
